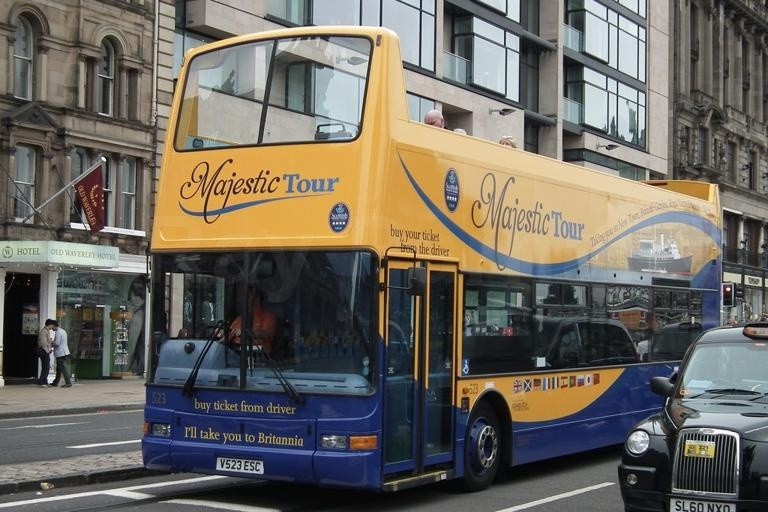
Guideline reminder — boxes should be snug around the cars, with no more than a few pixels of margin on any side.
[618,323,767,511]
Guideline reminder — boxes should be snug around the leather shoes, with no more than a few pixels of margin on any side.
[50,383,57,386]
[61,384,71,388]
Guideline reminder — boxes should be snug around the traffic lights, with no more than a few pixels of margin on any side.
[723,283,735,308]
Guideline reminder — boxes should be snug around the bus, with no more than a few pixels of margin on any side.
[141,24,725,497]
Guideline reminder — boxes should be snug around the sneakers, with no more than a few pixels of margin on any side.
[37,384,48,388]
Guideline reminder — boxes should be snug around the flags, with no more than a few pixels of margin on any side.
[74,164,105,233]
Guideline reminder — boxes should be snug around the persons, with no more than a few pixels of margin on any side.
[499,136,517,148]
[37,319,54,387]
[201,292,216,336]
[217,278,276,355]
[177,328,189,337]
[425,110,447,128]
[49,320,72,388]
[125,275,145,375]
[184,290,194,327]
[731,310,768,325]
